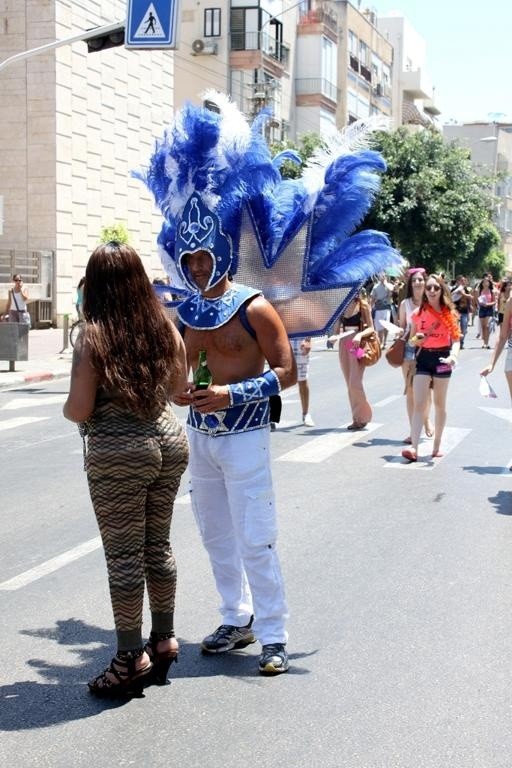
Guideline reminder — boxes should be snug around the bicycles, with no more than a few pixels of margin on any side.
[68,319,84,349]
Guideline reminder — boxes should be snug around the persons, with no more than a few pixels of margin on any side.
[170,231,298,675]
[74,276,88,320]
[3,274,28,322]
[63,241,191,697]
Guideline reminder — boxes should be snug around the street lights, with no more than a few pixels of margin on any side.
[467,136,502,148]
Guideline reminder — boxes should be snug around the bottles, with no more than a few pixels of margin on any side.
[192,348,214,410]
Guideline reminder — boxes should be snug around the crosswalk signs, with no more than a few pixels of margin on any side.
[123,0,175,51]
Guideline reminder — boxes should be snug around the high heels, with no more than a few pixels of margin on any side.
[88,632,179,697]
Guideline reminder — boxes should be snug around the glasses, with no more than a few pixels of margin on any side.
[427,285,440,290]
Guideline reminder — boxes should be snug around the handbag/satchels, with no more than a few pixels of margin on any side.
[357,332,381,366]
[386,339,404,367]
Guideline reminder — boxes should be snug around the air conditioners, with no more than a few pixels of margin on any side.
[189,35,218,56]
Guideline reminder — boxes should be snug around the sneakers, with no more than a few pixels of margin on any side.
[303,414,314,427]
[202,615,256,653]
[259,643,288,673]
[403,450,416,461]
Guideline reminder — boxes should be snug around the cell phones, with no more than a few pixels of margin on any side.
[416,332,424,338]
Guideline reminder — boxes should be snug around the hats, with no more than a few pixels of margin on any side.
[174,191,233,292]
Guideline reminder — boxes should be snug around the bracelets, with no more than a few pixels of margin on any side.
[228,370,281,407]
